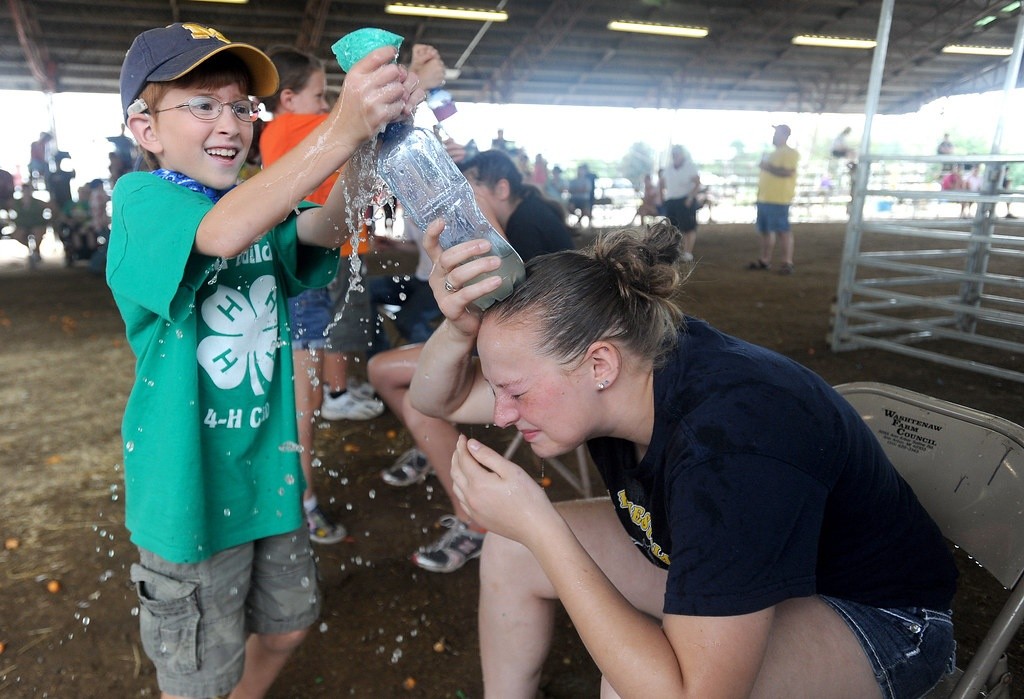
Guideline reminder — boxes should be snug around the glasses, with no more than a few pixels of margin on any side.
[151,97,261,121]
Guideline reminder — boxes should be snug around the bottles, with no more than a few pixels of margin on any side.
[377,122,525,311]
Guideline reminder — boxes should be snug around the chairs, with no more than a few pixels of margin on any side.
[604,379,1024,699]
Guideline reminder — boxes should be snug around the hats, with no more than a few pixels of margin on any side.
[772,124,791,136]
[120,21,280,123]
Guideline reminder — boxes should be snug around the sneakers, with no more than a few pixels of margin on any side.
[384,449,431,485]
[410,514,490,574]
[320,371,374,397]
[320,386,384,419]
[299,502,347,544]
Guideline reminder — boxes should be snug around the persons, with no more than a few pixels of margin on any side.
[259,41,452,545]
[408,214,960,699]
[626,168,665,226]
[107,22,445,699]
[105,124,135,170]
[108,151,123,188]
[432,124,598,229]
[944,162,1018,220]
[937,133,956,155]
[747,124,799,272]
[11,131,108,266]
[366,149,582,573]
[325,218,386,420]
[658,145,701,262]
[832,126,858,154]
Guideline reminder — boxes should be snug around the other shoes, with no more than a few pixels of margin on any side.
[681,253,693,262]
[746,259,771,269]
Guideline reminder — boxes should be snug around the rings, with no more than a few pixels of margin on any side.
[444,274,462,292]
